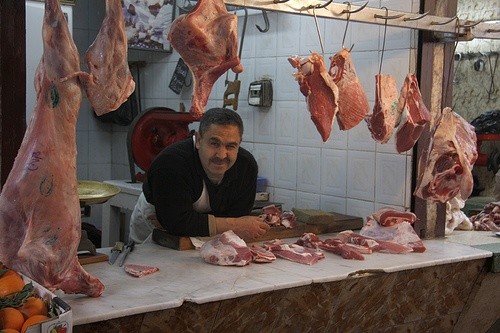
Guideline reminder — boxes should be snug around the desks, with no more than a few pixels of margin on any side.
[461,197,497,217]
[102,180,143,249]
[445,230,500,273]
[54,233,493,333]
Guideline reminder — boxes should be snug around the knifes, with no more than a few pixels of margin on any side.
[108,242,123,264]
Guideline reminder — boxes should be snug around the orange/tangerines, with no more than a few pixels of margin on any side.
[0,272,49,333]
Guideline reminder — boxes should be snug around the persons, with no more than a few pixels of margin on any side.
[129,109,270,244]
[124,0,172,50]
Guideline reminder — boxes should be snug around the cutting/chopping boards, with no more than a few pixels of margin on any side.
[153,210,364,250]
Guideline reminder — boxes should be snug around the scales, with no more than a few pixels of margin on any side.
[75,178,122,254]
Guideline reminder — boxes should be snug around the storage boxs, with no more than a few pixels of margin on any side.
[255,176,269,201]
[17,272,72,333]
